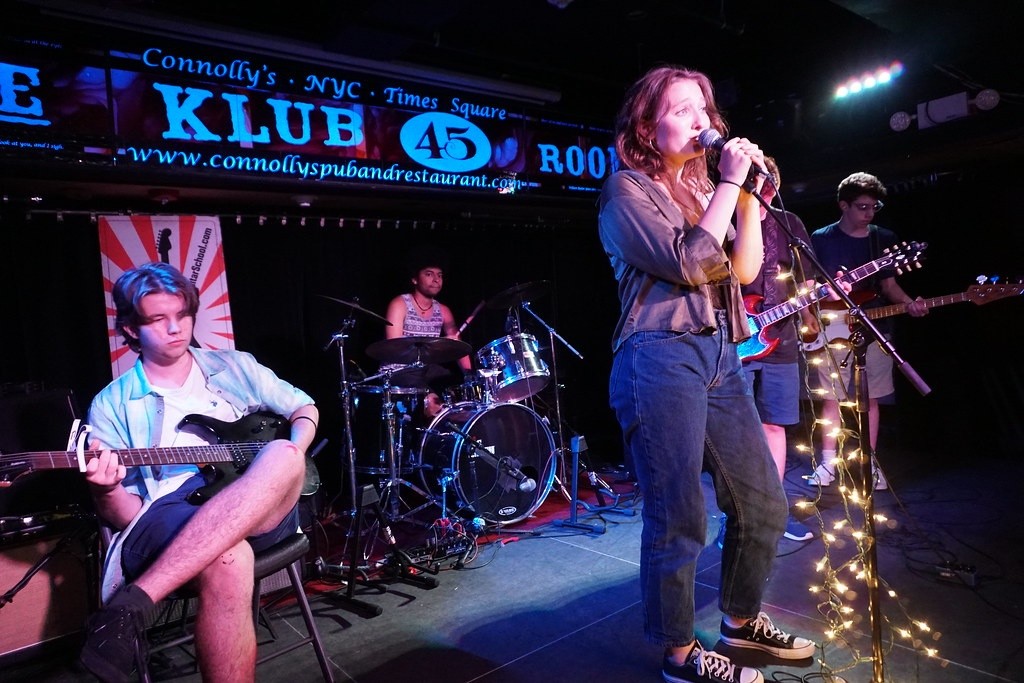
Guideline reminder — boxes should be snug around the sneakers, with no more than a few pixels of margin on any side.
[721,612,814,659]
[718,513,728,549]
[784,513,814,540]
[78,607,151,683]
[662,638,764,683]
[808,464,836,486]
[872,464,887,490]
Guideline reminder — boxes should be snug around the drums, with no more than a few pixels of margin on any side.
[473,332,552,402]
[342,384,428,475]
[442,378,488,405]
[420,401,557,524]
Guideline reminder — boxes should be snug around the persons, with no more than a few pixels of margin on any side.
[386,247,471,496]
[717,152,852,550]
[76,258,320,683]
[597,64,816,683]
[807,172,929,491]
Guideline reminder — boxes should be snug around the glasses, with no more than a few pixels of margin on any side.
[853,200,883,212]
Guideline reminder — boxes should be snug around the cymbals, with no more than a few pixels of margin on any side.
[485,279,554,310]
[318,293,393,326]
[366,336,473,363]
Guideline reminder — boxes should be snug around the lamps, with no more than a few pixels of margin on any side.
[889,88,1000,132]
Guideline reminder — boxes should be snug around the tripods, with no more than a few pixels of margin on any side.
[260,315,527,616]
[519,290,617,499]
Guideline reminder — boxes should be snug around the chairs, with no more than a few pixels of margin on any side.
[69,383,336,683]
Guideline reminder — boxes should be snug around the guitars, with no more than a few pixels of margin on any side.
[737,240,929,363]
[0,411,321,505]
[798,274,1024,352]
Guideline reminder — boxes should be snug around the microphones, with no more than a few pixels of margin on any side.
[513,468,537,492]
[699,129,765,176]
[505,308,515,332]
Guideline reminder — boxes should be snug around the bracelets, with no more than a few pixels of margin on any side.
[291,416,317,430]
[717,178,740,188]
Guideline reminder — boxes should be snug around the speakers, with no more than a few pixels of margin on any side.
[140,529,306,635]
[0,518,106,666]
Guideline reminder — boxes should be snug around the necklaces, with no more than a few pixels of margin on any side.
[411,292,432,315]
